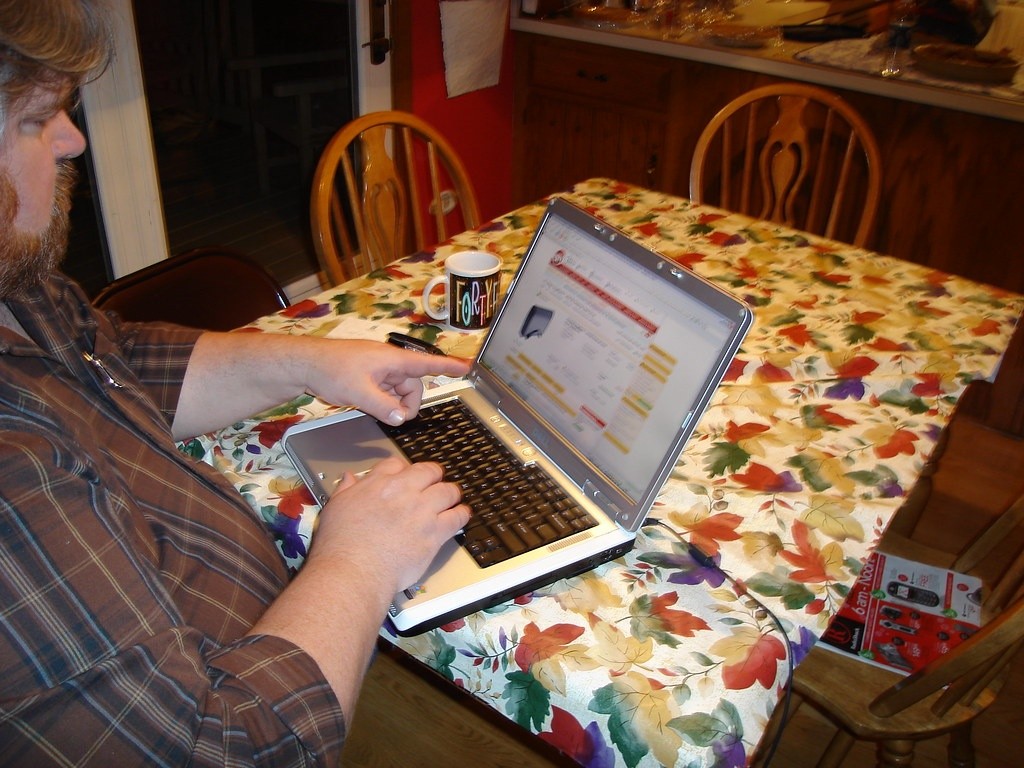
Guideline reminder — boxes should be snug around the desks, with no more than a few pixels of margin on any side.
[177,177,1024,768]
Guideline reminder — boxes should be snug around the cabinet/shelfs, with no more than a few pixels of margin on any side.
[510,29,1024,438]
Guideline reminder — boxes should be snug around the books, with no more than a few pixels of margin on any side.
[815,549,984,691]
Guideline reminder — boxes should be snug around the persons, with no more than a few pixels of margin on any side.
[0,0,471,768]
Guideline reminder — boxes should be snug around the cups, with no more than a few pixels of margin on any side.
[421,251,504,333]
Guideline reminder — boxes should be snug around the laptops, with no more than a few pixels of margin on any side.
[279,198,754,633]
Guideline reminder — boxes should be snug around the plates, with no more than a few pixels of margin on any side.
[705,30,780,49]
[912,42,1023,82]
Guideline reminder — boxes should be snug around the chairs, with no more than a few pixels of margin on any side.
[312,112,480,288]
[691,81,883,250]
[91,247,291,334]
[751,493,1024,768]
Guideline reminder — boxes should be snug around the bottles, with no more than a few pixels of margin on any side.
[878,0,918,79]
